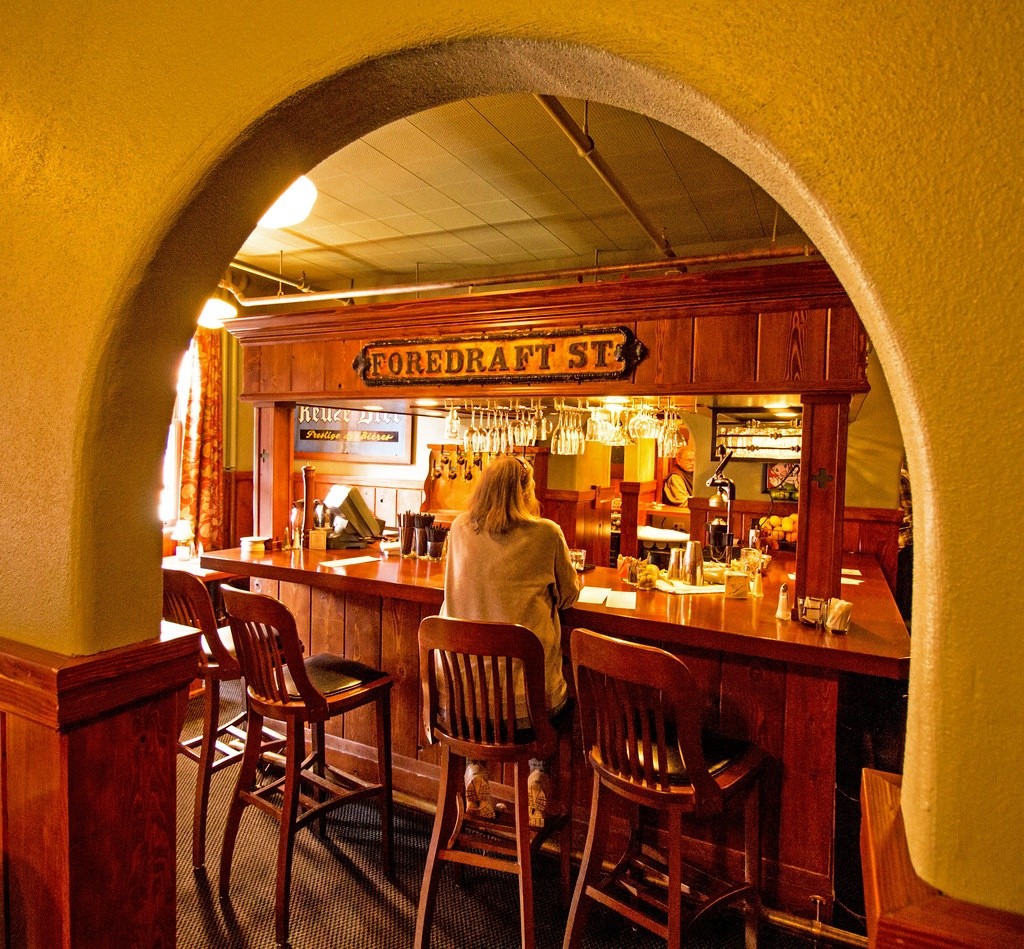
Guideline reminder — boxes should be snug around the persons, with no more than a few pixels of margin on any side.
[663,446,695,508]
[432,455,581,828]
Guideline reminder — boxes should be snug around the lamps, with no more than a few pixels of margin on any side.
[170,520,196,560]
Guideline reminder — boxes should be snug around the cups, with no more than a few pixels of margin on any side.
[427,541,444,561]
[398,526,415,557]
[617,557,657,590]
[175,546,190,560]
[413,527,428,557]
[569,549,586,570]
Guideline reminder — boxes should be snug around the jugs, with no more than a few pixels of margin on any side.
[668,540,705,586]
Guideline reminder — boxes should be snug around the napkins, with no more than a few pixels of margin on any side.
[840,568,862,576]
[318,555,383,568]
[841,577,865,586]
[787,572,796,580]
[578,585,612,605]
[606,591,637,610]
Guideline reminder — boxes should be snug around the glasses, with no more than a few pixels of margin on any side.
[514,457,527,480]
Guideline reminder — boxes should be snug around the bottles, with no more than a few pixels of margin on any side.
[774,583,791,620]
[741,547,758,571]
[753,569,764,597]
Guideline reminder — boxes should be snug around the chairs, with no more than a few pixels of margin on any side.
[563,626,773,949]
[412,614,574,949]
[160,568,309,870]
[218,583,398,945]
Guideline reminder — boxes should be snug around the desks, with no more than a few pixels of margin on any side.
[162,555,249,625]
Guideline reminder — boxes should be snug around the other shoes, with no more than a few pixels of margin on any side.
[463,764,496,819]
[528,769,550,827]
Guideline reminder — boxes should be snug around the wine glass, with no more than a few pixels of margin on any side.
[442,401,687,459]
